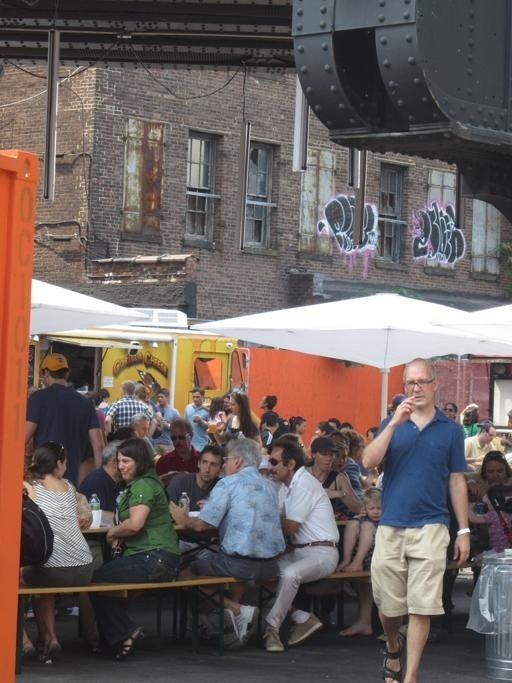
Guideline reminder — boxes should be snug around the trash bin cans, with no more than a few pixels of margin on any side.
[481,547,512,681]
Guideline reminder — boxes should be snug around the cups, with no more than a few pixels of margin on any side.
[91,510,102,528]
[159,418,167,432]
[207,419,217,433]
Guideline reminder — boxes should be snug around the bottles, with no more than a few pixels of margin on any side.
[89,493,101,509]
[238,431,245,439]
[180,491,190,519]
[115,491,124,524]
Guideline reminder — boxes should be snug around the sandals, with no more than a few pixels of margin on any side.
[381,638,403,683]
[39,640,61,664]
[22,640,36,659]
[115,626,144,659]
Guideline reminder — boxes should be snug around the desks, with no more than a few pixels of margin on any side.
[79,518,349,645]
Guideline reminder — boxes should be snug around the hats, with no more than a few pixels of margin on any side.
[311,437,339,453]
[40,353,69,371]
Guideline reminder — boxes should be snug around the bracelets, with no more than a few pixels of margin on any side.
[457,528,470,536]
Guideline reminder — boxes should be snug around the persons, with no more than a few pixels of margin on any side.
[476,450,512,501]
[169,437,286,652]
[89,440,180,661]
[263,439,340,652]
[336,488,382,572]
[468,481,512,553]
[17,353,512,662]
[18,439,93,660]
[361,357,472,683]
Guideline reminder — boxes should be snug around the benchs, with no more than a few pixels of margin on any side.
[14,560,476,674]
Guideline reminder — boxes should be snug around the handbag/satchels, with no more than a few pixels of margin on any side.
[20,495,54,567]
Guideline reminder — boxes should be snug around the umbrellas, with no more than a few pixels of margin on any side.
[189,291,512,421]
[31,279,150,338]
[471,302,512,337]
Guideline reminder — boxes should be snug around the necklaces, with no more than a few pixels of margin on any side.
[312,467,329,482]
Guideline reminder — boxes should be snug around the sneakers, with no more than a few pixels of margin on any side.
[265,628,285,652]
[187,604,259,650]
[288,612,323,646]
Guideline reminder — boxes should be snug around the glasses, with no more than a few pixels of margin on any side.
[170,434,186,440]
[406,377,434,388]
[270,458,283,465]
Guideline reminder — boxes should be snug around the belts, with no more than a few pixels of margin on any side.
[293,541,339,548]
